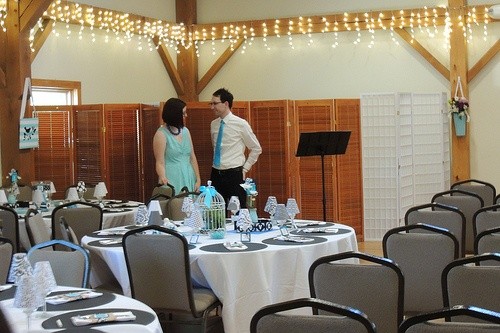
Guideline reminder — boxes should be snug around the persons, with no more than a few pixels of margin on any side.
[153,98,201,197]
[209,88,262,217]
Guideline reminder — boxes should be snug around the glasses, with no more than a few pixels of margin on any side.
[208,102,226,107]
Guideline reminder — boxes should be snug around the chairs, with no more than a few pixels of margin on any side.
[0,181,500,333]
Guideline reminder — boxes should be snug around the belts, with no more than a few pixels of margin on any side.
[212,167,242,174]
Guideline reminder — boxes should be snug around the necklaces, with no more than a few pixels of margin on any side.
[166,125,180,135]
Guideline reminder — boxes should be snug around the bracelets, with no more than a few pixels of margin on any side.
[196,179,201,182]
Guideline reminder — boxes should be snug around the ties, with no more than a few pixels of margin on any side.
[214,120,224,166]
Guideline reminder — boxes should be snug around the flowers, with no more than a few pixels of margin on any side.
[447,96,473,122]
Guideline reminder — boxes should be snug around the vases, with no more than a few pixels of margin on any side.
[452,112,468,138]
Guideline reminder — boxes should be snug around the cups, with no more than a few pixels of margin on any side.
[8,253,57,308]
[0,182,108,208]
[134,196,300,230]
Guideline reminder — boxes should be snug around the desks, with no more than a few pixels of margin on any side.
[0,283,164,333]
[3,197,147,252]
[80,217,359,333]
